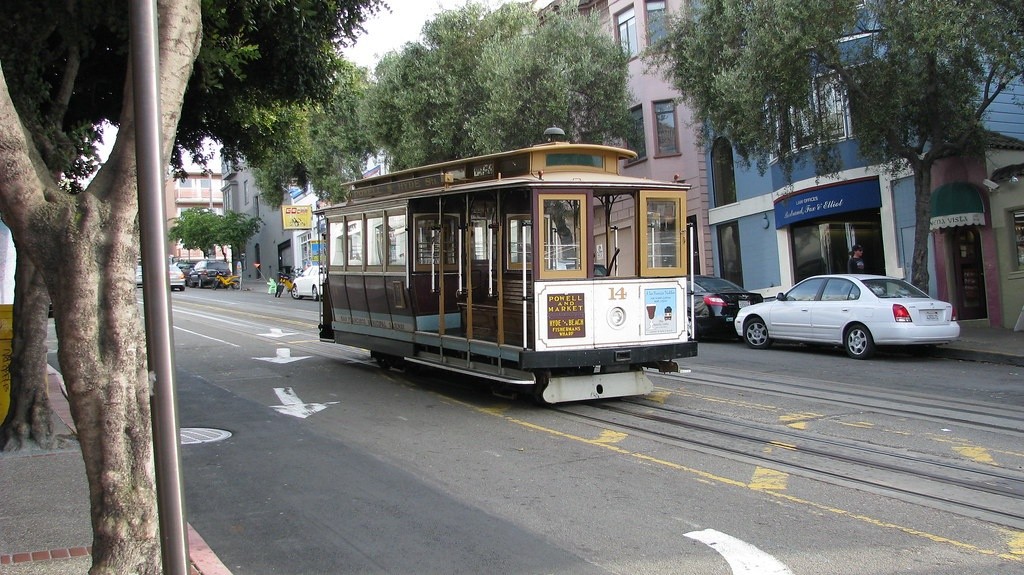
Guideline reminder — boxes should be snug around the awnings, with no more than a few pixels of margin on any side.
[927,181,986,233]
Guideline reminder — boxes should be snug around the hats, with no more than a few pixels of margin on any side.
[852,244,864,252]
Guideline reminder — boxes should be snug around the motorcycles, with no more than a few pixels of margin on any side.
[213,270,241,290]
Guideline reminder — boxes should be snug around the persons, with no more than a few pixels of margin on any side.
[267,272,288,298]
[847,244,865,274]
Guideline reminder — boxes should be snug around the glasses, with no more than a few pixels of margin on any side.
[857,249,863,251]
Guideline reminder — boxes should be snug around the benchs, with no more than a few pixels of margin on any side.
[456,270,531,339]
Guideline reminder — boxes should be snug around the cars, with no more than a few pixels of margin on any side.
[685,273,764,342]
[186,260,231,289]
[136,260,143,287]
[291,265,327,301]
[170,264,186,292]
[734,271,961,360]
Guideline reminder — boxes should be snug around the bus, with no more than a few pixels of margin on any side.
[312,121,700,410]
